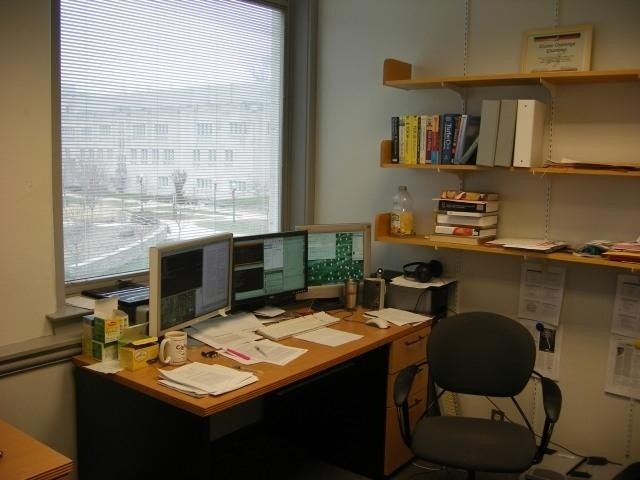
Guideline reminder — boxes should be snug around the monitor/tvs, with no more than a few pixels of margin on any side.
[149,232,234,337]
[225,229,308,318]
[295,222,372,310]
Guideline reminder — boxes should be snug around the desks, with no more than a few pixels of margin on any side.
[70,308,434,479]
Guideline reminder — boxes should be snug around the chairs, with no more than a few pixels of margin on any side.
[393,311,565,480]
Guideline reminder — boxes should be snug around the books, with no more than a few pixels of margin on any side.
[429,189,499,247]
[156,362,260,399]
[601,240,640,263]
[391,114,479,165]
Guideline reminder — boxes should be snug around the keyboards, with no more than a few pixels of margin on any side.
[255,310,341,342]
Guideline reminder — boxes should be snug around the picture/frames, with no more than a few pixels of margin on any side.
[519,23,594,72]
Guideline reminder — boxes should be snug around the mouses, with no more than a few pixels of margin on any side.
[366,317,391,330]
[582,245,604,255]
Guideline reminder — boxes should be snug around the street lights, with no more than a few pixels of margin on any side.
[137,174,145,211]
[230,183,239,227]
[213,179,218,215]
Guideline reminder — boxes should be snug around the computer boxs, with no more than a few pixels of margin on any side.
[371,270,448,328]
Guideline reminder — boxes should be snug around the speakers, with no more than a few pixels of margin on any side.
[362,278,385,312]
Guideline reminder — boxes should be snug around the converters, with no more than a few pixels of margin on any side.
[585,456,609,465]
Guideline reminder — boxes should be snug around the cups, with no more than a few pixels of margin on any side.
[343,278,360,309]
[159,330,188,365]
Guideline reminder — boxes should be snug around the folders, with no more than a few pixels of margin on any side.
[476,100,547,168]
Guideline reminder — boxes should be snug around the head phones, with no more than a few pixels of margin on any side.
[402,260,443,283]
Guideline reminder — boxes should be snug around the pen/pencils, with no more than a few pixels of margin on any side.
[227,349,250,360]
[254,346,268,359]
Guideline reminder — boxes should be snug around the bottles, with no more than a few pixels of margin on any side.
[389,186,419,236]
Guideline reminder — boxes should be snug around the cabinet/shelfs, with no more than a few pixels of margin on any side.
[378,328,429,475]
[374,58,640,272]
